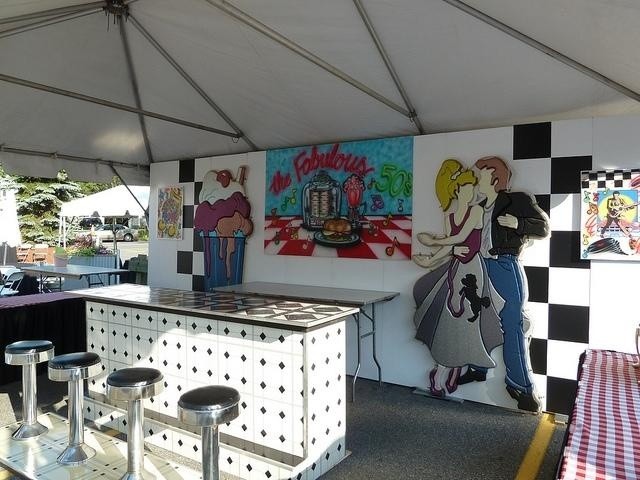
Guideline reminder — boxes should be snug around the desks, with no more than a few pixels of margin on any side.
[21,264,129,294]
[210,281,400,403]
[553,349,639,479]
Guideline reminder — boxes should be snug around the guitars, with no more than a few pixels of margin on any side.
[609,202,639,218]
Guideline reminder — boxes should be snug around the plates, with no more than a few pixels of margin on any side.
[314,232,359,247]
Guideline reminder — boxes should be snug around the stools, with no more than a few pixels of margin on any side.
[48,352,104,466]
[106,367,164,479]
[177,385,242,479]
[4,340,55,441]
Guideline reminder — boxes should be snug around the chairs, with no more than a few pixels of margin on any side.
[0,271,26,297]
[2,269,20,289]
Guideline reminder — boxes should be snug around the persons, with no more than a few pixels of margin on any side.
[410,156,552,412]
[600,191,632,238]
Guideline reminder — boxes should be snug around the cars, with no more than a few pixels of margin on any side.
[80,223,138,241]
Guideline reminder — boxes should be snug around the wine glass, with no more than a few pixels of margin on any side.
[347,189,362,222]
